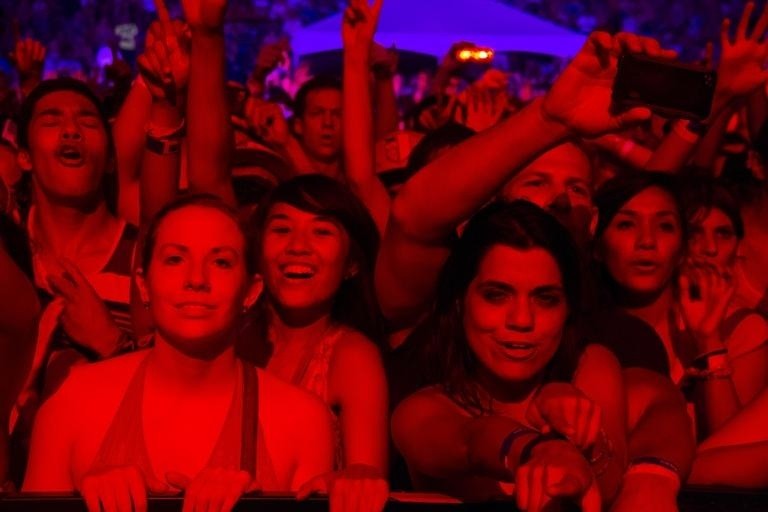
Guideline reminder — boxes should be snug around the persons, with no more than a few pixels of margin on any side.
[0,1,768,512]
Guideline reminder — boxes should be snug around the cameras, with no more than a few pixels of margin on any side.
[614,55,717,119]
